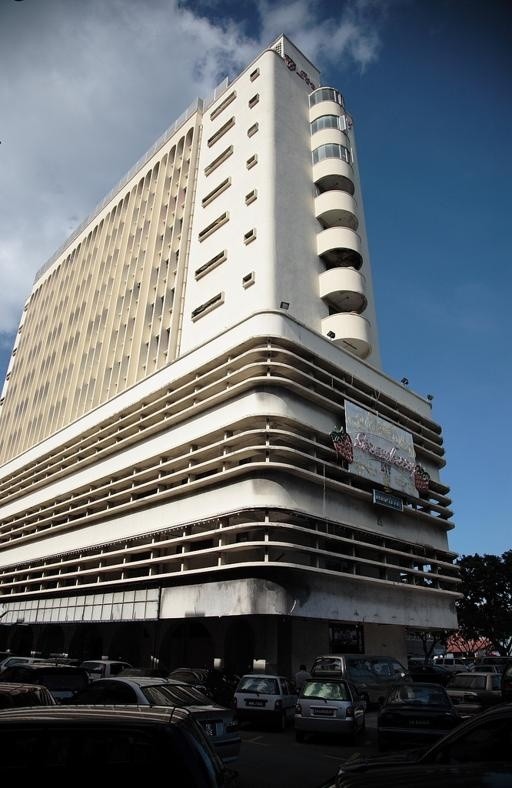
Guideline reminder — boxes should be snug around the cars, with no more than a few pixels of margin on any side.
[232,674,299,729]
[376,681,461,752]
[408,650,512,718]
[0,648,241,787]
[294,677,368,741]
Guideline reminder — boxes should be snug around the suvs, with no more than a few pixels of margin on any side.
[326,702,512,787]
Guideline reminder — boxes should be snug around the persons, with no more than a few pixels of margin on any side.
[295,664,313,698]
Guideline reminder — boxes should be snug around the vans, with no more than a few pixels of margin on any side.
[311,654,410,703]
